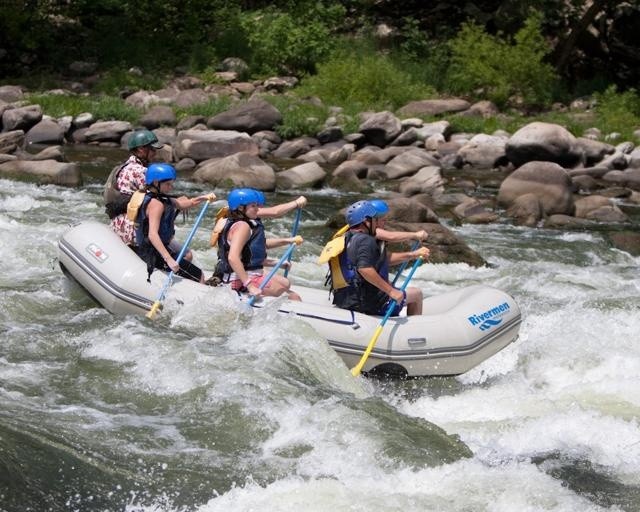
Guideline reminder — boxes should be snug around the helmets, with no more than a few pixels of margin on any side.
[258,191,265,204]
[227,188,259,210]
[345,200,377,227]
[170,166,176,180]
[128,130,164,150]
[370,200,389,216]
[145,164,175,185]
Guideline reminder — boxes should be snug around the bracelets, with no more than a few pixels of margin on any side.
[163,256,172,261]
[294,199,299,208]
[242,278,250,288]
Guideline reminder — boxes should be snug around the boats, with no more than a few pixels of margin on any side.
[58,219,522,383]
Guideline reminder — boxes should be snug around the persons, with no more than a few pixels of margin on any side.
[218,191,303,300]
[108,128,164,253]
[370,197,430,255]
[133,163,217,285]
[317,199,431,316]
[242,188,303,290]
